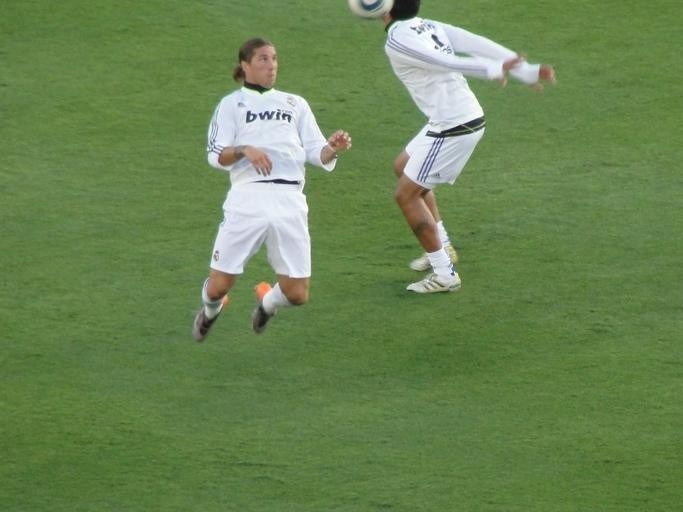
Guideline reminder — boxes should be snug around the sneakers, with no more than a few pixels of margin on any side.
[410,246,459,272]
[193,295,230,343]
[406,271,463,294]
[251,282,276,333]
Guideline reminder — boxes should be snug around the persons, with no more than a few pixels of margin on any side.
[379,1,557,294]
[191,36,356,345]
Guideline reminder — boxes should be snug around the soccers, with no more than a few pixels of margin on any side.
[347,0,391,20]
